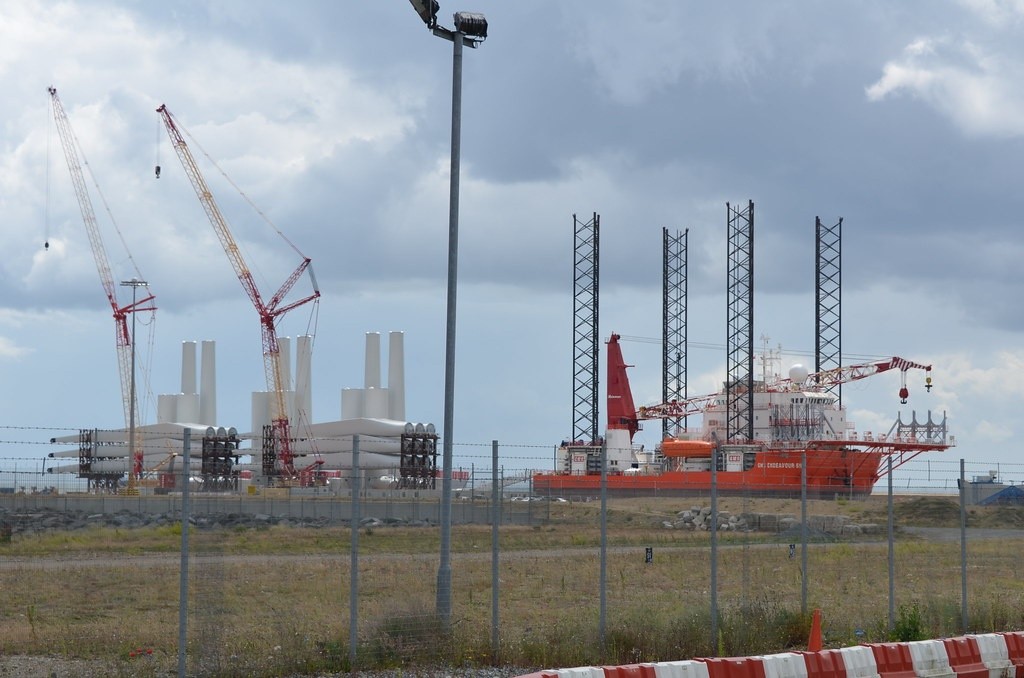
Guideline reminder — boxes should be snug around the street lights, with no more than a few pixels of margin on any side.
[409,0,491,641]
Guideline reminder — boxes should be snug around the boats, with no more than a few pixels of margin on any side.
[529,200,959,498]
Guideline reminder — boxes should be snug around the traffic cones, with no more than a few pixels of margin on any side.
[807,608,823,653]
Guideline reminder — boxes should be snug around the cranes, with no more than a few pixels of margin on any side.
[147,102,344,485]
[47,83,177,491]
